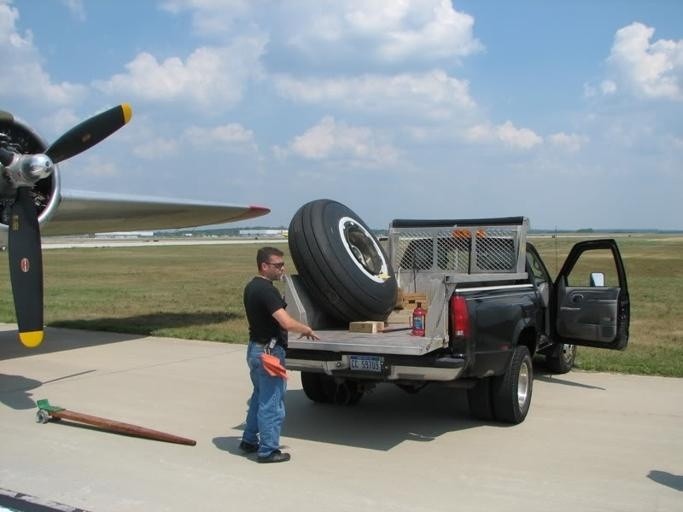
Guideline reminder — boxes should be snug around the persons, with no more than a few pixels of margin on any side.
[237,246,318,463]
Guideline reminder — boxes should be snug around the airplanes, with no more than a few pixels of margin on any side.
[0,100,272,349]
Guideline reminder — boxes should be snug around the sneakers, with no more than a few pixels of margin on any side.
[256,449,291,463]
[239,441,259,453]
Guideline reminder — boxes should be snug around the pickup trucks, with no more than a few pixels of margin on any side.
[279,214,631,426]
